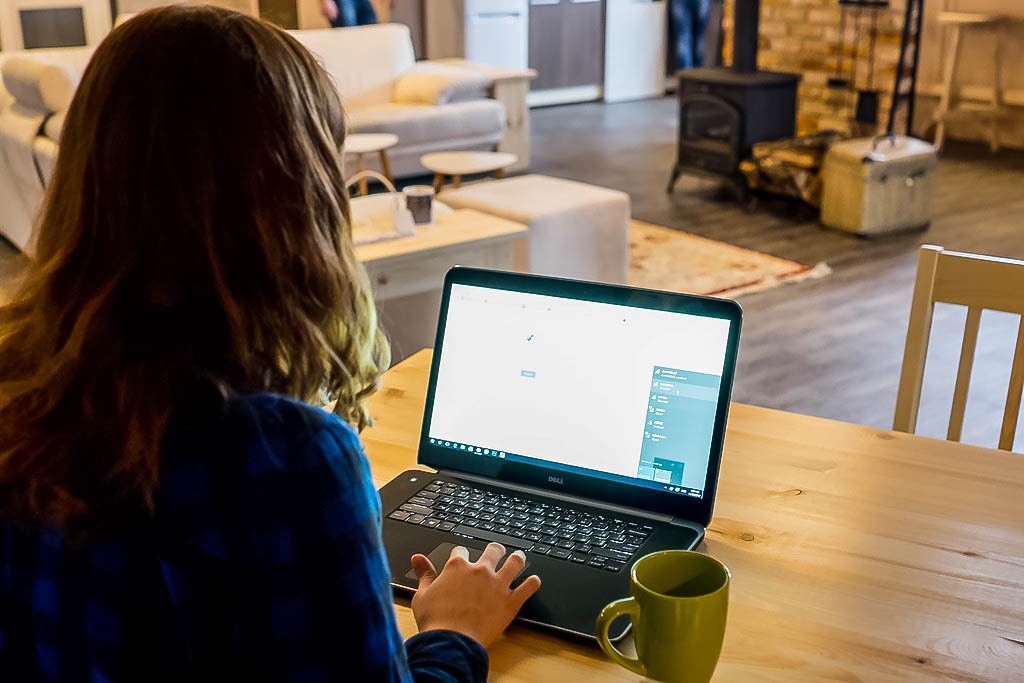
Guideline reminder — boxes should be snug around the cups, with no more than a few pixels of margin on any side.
[402,184,435,224]
[595,550,732,683]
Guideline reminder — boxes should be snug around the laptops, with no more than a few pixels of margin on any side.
[376,264,742,648]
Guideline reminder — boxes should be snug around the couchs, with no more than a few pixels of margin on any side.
[0,23,538,250]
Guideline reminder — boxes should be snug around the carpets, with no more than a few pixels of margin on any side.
[626,219,833,296]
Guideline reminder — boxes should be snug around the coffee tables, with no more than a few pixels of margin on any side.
[356,205,531,370]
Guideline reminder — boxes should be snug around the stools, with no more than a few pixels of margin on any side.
[421,150,517,195]
[934,9,1007,150]
[442,167,631,289]
[338,133,400,197]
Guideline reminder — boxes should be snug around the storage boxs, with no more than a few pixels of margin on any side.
[819,131,942,236]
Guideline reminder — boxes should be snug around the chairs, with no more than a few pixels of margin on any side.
[888,244,1023,453]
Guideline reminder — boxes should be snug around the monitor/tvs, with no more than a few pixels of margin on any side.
[20,8,85,49]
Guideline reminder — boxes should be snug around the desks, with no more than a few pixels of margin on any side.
[316,347,1023,683]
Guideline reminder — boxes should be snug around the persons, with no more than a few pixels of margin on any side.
[671,1,711,77]
[320,0,377,28]
[2,9,544,683]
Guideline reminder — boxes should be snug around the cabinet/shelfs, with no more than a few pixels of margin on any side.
[664,61,799,208]
